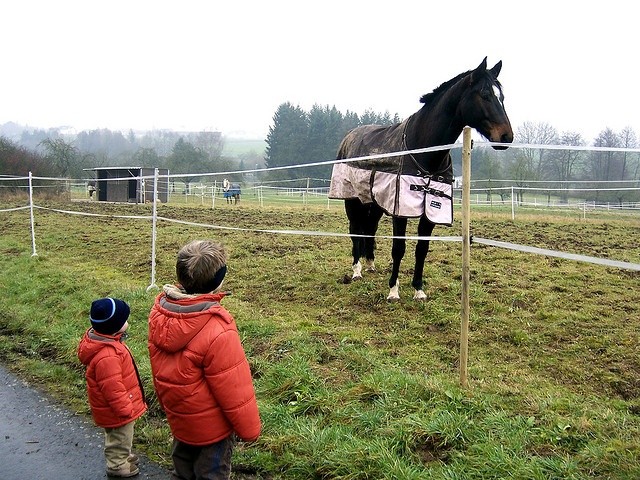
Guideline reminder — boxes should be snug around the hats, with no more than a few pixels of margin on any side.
[91,298,131,334]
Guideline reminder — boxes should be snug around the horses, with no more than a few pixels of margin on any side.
[336,56,514,300]
[222,178,241,205]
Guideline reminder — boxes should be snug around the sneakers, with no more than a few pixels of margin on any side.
[107,454,140,478]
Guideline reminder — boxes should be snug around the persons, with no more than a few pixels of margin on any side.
[78,298,147,478]
[149,240,261,480]
